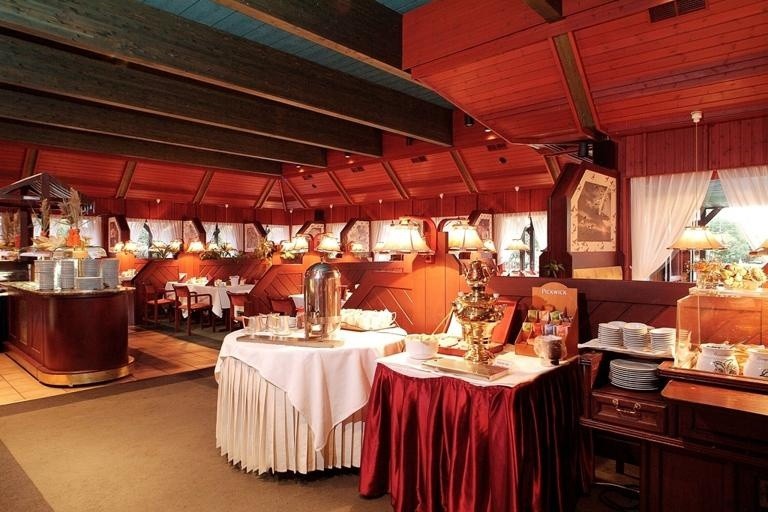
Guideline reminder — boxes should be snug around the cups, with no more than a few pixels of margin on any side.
[339,308,396,330]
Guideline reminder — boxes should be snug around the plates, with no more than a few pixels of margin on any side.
[289,317,295,327]
[597,319,677,353]
[34,258,120,291]
[609,357,658,392]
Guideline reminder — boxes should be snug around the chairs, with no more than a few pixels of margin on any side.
[140,279,298,337]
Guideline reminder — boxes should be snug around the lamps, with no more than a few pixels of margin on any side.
[382,222,431,257]
[448,218,483,253]
[666,224,728,284]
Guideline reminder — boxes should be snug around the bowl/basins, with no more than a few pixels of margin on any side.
[405,334,439,359]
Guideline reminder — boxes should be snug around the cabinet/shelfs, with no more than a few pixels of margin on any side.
[579,346,767,511]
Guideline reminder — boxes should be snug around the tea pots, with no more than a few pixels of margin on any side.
[242,314,289,336]
